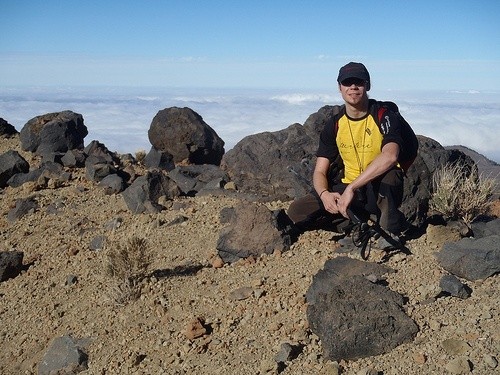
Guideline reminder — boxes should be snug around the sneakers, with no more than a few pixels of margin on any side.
[380,232,401,250]
[339,214,376,246]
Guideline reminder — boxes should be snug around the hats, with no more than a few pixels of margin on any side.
[337,62,370,81]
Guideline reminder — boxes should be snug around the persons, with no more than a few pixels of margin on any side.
[287,62,419,251]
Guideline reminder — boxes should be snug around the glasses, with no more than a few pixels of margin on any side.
[340,78,368,86]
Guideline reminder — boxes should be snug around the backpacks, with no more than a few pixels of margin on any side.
[385,101,419,174]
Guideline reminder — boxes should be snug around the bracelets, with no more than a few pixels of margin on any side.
[319,190,328,198]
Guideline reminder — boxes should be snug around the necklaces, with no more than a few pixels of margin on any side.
[346,113,367,175]
